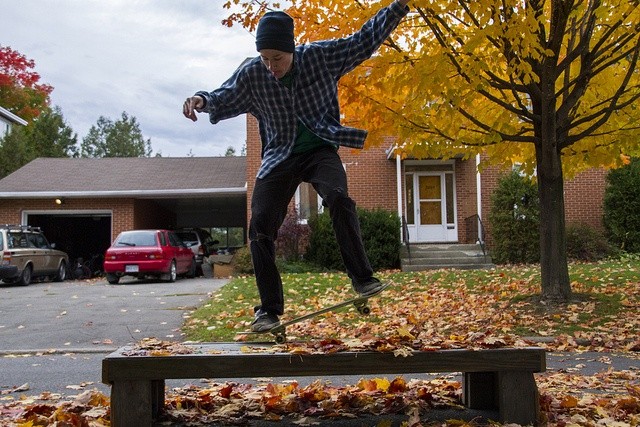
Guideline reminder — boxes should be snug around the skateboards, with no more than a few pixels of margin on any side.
[236,280,393,343]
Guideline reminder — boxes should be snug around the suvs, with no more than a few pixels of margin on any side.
[175,228,220,263]
[0,224,69,285]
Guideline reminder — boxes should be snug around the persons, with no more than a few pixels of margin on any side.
[182,1,410,331]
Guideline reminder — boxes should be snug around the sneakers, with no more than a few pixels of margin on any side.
[352,277,382,295]
[251,311,280,331]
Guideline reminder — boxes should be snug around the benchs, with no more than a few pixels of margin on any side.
[102,338,545,427]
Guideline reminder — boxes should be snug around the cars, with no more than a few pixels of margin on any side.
[103,228,197,284]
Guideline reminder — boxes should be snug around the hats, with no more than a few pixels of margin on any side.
[256,12,295,52]
